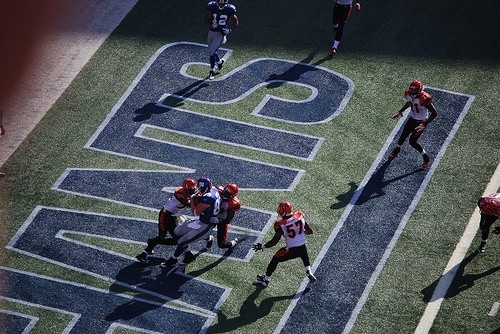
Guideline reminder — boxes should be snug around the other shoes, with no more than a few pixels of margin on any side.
[306,270,316,281]
[179,250,196,266]
[235,237,238,246]
[206,235,213,248]
[478,239,488,253]
[257,274,270,286]
[389,146,400,157]
[218,59,224,71]
[160,254,178,268]
[422,153,429,168]
[148,238,154,254]
[209,68,215,80]
[331,47,337,56]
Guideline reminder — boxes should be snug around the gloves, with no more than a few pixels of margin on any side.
[185,209,193,216]
[222,28,232,34]
[251,242,265,252]
[415,123,425,132]
[480,221,483,230]
[210,217,219,223]
[391,112,400,119]
[354,3,360,10]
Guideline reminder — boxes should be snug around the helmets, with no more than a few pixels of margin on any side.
[224,184,238,200]
[182,178,197,193]
[481,199,499,214]
[409,79,423,95]
[198,177,212,195]
[216,0,228,10]
[277,199,294,218]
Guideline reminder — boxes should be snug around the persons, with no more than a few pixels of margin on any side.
[328,0,361,57]
[203,183,241,250]
[160,177,221,267]
[251,200,317,287]
[207,0,238,79]
[389,79,437,166]
[135,178,198,265]
[477,193,500,253]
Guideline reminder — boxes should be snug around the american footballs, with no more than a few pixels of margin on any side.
[196,203,210,215]
